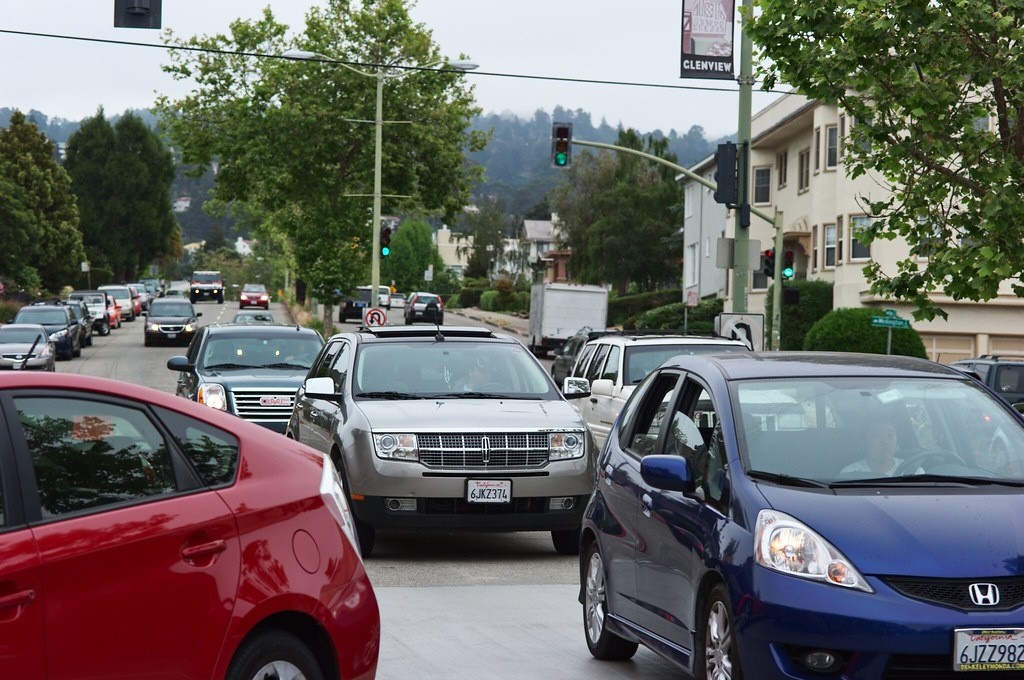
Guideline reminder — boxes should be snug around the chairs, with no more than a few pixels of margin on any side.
[209,342,234,366]
[80,435,146,510]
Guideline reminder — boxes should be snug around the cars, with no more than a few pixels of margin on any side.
[576,351,1024,680]
[338,287,372,323]
[390,293,406,309]
[0,372,382,680]
[141,297,204,349]
[126,286,141,316]
[29,301,94,347]
[97,285,135,322]
[106,297,123,329]
[237,283,271,310]
[404,292,428,316]
[0,325,55,372]
[126,284,151,312]
[68,291,110,335]
[14,305,81,361]
[378,287,391,311]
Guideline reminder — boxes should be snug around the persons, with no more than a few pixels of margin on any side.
[455,366,483,392]
[280,341,310,366]
[837,419,925,479]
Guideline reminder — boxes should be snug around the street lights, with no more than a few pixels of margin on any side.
[281,48,478,310]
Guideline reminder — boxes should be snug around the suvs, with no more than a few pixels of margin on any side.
[405,293,444,326]
[950,352,1024,415]
[551,327,622,392]
[562,329,749,453]
[166,322,327,436]
[285,326,599,556]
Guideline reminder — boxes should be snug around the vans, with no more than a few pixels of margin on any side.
[187,270,226,304]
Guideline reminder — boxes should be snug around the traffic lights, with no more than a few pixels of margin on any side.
[791,270,792,271]
[380,227,391,259]
[763,248,775,280]
[782,248,794,281]
[550,121,574,168]
[712,141,735,204]
